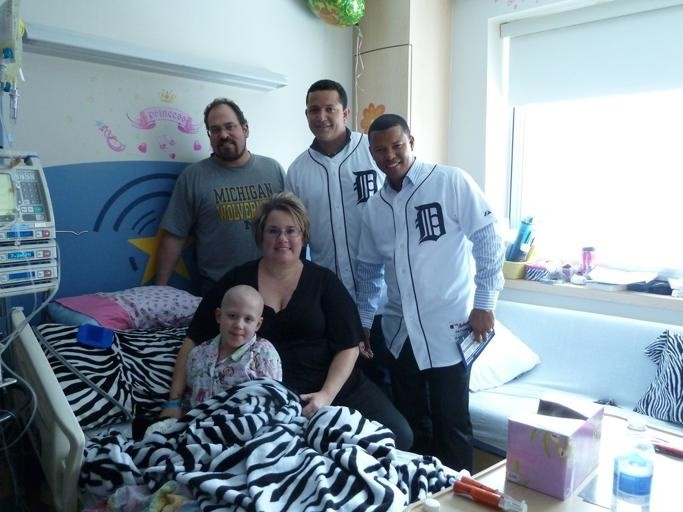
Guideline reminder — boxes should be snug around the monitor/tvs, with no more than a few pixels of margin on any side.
[0,172,21,224]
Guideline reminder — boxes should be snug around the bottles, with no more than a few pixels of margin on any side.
[582,246,595,277]
[612,411,655,512]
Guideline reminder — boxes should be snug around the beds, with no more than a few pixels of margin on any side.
[6,160,471,512]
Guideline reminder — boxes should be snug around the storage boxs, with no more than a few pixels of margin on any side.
[505,396,603,498]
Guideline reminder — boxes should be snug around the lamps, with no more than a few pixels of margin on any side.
[22,22,289,93]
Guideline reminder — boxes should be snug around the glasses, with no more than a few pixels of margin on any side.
[207,124,239,137]
[265,226,302,238]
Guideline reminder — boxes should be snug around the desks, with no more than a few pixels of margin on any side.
[405,458,682,511]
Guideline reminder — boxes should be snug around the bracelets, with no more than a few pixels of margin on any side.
[162,399,183,411]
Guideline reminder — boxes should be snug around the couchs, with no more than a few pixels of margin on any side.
[469,300,683,458]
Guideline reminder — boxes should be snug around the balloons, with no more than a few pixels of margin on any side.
[303,0,367,28]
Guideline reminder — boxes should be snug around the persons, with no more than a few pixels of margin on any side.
[287,80,394,395]
[161,191,413,452]
[185,285,283,411]
[353,113,507,477]
[152,98,288,295]
